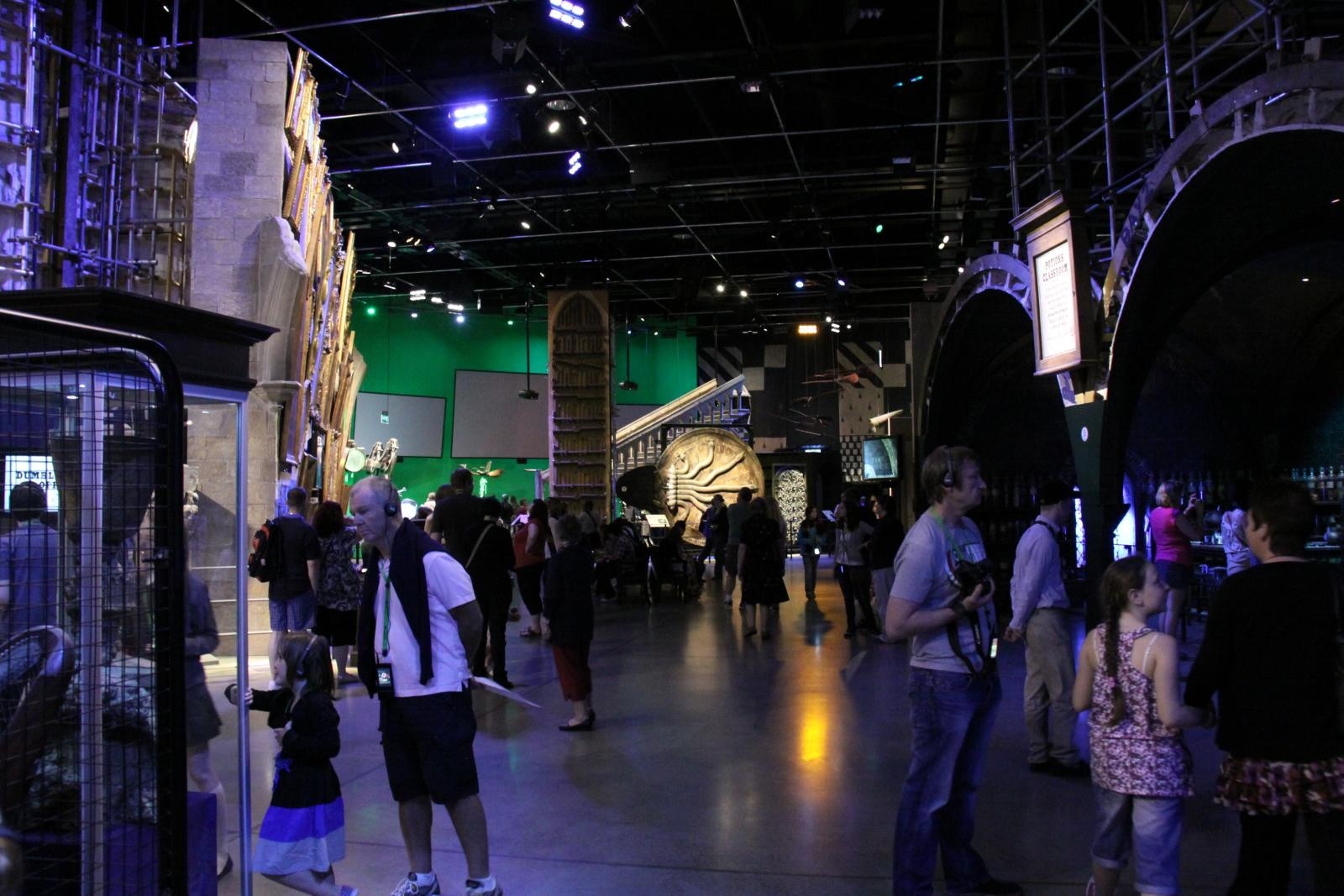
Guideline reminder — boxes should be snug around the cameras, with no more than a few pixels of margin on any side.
[954,562,991,597]
[1196,499,1202,505]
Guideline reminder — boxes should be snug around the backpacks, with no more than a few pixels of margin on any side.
[248,519,283,583]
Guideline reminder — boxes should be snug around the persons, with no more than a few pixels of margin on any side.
[350,477,504,896]
[1003,480,1089,776]
[661,521,705,602]
[310,501,363,683]
[798,492,906,643]
[698,486,789,640]
[885,444,1024,896]
[258,488,319,684]
[1149,481,1204,661]
[225,631,358,896]
[1220,477,1254,575]
[1185,479,1344,896]
[416,470,638,731]
[0,481,73,638]
[1071,556,1203,896]
[118,528,233,881]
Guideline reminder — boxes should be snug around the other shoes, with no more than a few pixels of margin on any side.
[1055,762,1091,779]
[947,876,1024,896]
[862,621,872,636]
[869,635,885,644]
[519,626,542,637]
[1029,758,1057,774]
[340,885,353,896]
[761,633,771,640]
[508,613,521,621]
[725,600,733,607]
[338,674,358,685]
[1086,877,1096,896]
[559,710,596,732]
[216,852,233,882]
[806,591,816,599]
[493,677,514,689]
[844,629,855,638]
[744,628,757,639]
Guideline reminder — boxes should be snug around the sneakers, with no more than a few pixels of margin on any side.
[391,872,441,896]
[465,876,503,896]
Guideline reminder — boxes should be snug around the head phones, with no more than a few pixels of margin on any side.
[941,446,955,487]
[296,634,318,677]
[385,482,398,517]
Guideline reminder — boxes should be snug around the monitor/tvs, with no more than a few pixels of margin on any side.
[863,434,904,482]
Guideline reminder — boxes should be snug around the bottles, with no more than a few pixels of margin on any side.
[981,475,1043,547]
[1171,470,1228,508]
[1315,514,1344,545]
[1290,465,1343,501]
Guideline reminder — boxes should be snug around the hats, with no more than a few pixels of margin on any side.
[1032,479,1084,504]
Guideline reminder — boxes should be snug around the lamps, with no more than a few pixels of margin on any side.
[524,71,598,176]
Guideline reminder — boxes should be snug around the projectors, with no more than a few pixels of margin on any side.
[619,381,637,390]
[519,389,538,401]
